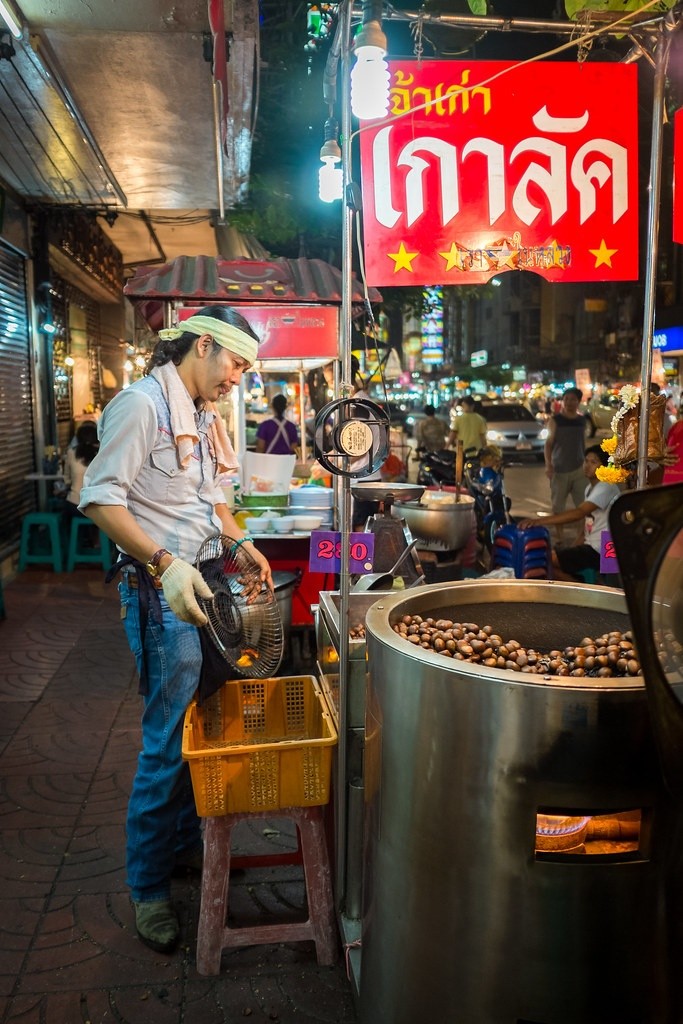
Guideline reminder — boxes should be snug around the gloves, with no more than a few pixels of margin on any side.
[160,556,214,628]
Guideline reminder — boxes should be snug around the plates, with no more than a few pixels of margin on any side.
[288,485,334,522]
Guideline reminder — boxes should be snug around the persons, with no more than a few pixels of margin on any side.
[64,421,100,548]
[544,388,587,545]
[444,395,488,457]
[77,305,274,951]
[415,405,456,452]
[518,444,620,554]
[257,394,298,455]
[524,396,562,416]
[310,354,382,485]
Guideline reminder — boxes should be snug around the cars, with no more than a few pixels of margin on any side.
[585,391,635,439]
[450,400,549,464]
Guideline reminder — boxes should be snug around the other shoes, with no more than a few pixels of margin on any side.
[163,844,203,867]
[130,892,179,949]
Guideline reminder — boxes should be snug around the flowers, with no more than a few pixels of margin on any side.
[595,384,637,485]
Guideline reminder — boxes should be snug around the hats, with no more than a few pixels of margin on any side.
[584,444,609,467]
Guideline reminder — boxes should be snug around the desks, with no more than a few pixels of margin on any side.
[26,464,65,479]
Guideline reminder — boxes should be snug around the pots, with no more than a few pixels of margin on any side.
[391,490,475,551]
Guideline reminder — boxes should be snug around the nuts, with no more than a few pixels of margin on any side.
[393,615,643,678]
[349,623,367,639]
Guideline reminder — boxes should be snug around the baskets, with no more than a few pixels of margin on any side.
[181,675,337,817]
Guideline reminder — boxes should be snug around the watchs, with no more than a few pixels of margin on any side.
[146,549,172,576]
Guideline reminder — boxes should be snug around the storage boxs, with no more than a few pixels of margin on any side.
[183,675,337,816]
[320,673,339,731]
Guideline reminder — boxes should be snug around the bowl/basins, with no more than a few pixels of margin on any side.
[241,492,287,517]
[244,518,268,533]
[271,518,293,533]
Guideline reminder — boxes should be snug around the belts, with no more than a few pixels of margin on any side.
[120,574,162,590]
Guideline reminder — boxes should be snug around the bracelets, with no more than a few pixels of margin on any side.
[231,537,253,553]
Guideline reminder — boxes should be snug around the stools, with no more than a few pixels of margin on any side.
[18,509,65,572]
[197,804,337,975]
[66,515,113,572]
[491,521,553,583]
